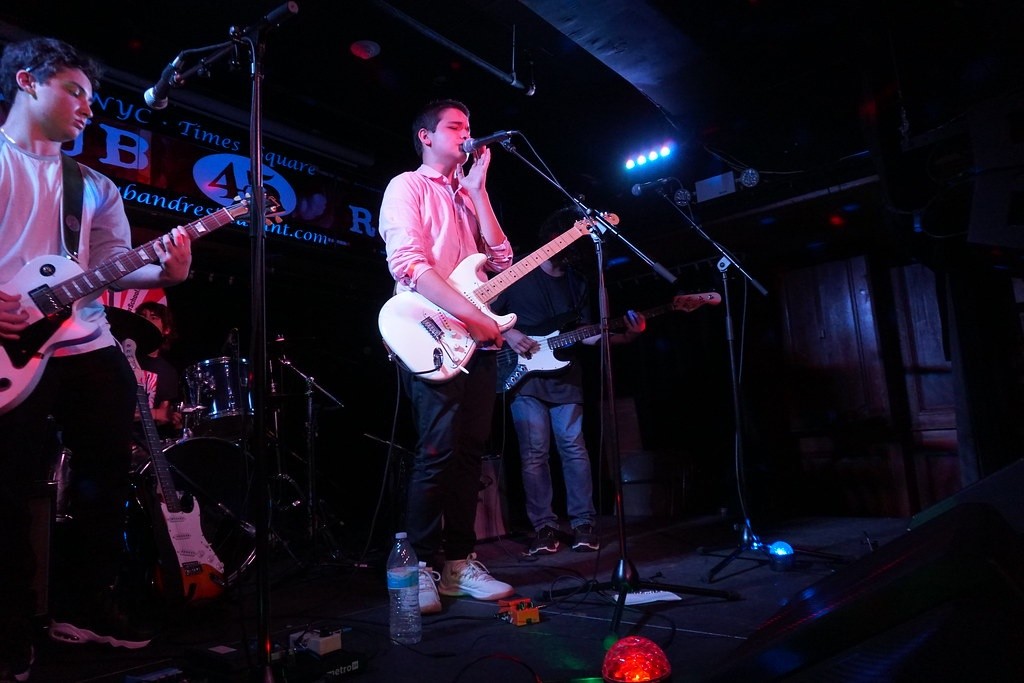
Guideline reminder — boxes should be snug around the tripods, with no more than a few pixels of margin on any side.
[498,140,852,637]
[268,335,345,561]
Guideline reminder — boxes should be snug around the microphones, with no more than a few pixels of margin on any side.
[462,131,519,153]
[221,326,238,356]
[143,52,185,111]
[240,520,257,535]
[632,177,672,196]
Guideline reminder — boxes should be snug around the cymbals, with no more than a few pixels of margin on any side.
[103,304,164,356]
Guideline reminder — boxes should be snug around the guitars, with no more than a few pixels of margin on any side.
[0,193,287,418]
[121,339,225,611]
[496,288,722,394]
[377,212,620,386]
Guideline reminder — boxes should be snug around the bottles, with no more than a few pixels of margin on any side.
[387,533,422,645]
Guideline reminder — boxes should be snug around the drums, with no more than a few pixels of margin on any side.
[126,435,272,586]
[182,355,281,435]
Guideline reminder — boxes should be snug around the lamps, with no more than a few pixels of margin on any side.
[674,169,761,208]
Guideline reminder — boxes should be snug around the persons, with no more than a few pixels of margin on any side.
[376,101,514,613]
[0,37,192,649]
[136,301,177,423]
[495,224,645,554]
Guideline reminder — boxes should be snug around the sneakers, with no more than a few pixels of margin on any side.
[530,526,560,555]
[438,551,512,601]
[0,643,35,681]
[49,600,152,649]
[571,522,601,551]
[400,561,440,612]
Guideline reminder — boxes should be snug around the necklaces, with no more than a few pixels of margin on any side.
[0,127,16,144]
